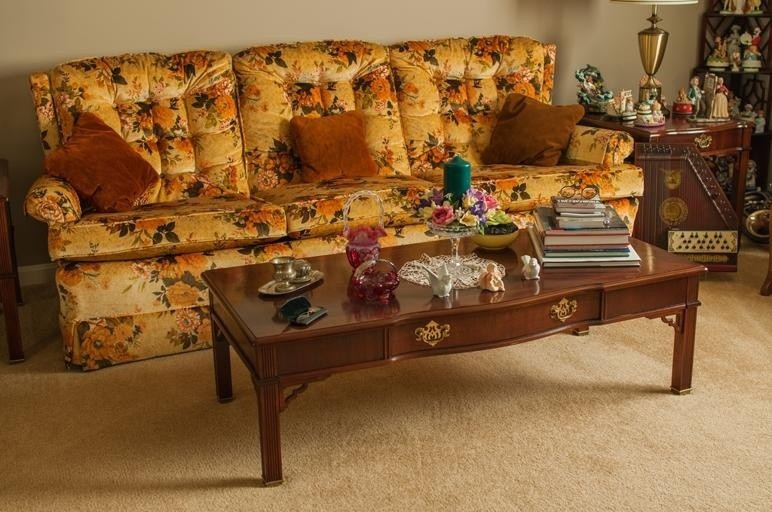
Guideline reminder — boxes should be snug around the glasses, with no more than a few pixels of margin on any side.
[298,306,321,323]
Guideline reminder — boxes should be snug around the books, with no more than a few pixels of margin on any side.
[528,195,642,268]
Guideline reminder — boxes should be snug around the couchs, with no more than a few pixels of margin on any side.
[24,35,644,372]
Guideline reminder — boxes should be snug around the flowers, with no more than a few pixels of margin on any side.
[418,188,510,231]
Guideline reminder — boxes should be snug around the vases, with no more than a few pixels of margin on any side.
[428,227,479,274]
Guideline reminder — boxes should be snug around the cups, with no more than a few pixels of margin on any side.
[291,258,312,284]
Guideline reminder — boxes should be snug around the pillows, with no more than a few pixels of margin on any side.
[290,111,377,183]
[484,93,585,167]
[44,112,159,213]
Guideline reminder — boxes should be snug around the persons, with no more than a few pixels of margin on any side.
[575,0,772,195]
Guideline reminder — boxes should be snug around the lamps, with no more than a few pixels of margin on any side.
[610,1,698,116]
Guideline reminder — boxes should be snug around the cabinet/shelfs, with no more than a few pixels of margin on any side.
[697,0,772,187]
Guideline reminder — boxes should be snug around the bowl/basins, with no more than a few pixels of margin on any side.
[746,209,771,238]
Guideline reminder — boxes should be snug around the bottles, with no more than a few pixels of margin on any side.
[442,152,473,203]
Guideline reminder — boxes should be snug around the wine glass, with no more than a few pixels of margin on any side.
[269,255,296,291]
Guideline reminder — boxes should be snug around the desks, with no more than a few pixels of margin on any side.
[576,106,755,272]
[0,160,26,363]
